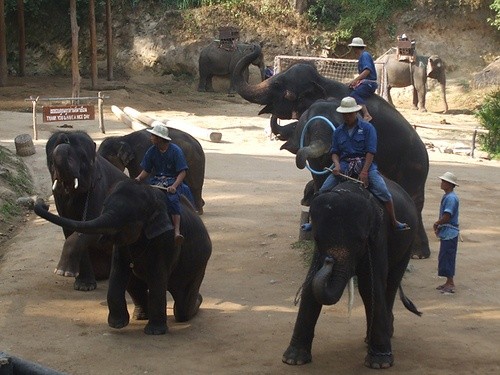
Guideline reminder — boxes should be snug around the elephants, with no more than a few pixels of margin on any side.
[377,53,448,114]
[281,174,418,369]
[34,126,213,335]
[198,41,265,93]
[234,46,431,259]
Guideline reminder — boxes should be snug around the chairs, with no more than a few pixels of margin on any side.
[218,26,239,39]
[397,35,415,56]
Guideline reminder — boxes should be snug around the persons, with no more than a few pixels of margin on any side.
[433,171,461,293]
[347,37,378,122]
[134,125,189,241]
[300,96,411,232]
[265,64,274,80]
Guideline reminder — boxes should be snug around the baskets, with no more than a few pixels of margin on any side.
[15,134,36,156]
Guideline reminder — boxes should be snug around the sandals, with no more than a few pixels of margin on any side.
[301,223,312,231]
[393,222,410,231]
[435,285,456,293]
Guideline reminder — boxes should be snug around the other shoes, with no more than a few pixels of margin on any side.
[363,114,373,121]
[175,235,184,247]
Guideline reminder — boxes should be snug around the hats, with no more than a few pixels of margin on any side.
[438,172,459,186]
[146,125,172,140]
[348,37,367,47]
[336,97,362,113]
